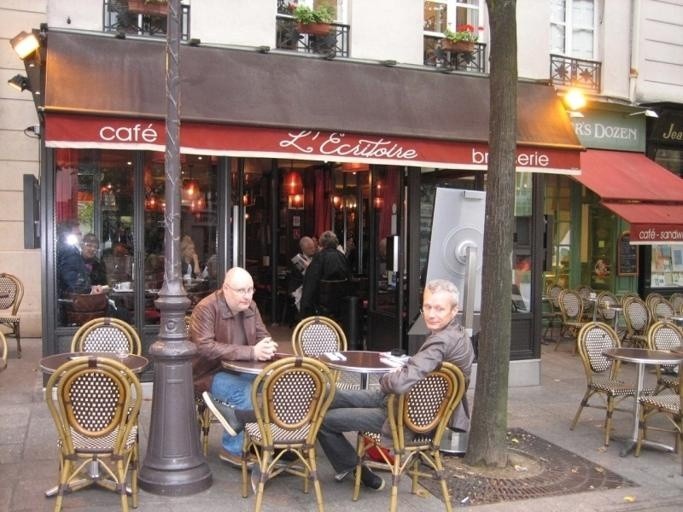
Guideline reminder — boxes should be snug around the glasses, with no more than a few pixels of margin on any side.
[226,285,256,294]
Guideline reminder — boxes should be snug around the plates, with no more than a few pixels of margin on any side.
[112,288,133,293]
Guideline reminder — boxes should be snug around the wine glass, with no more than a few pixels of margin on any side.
[114,348,128,364]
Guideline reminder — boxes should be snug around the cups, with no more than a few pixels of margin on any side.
[590,292,596,298]
[182,274,192,287]
[117,281,130,290]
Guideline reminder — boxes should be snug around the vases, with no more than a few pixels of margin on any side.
[128,0,170,17]
[441,38,475,53]
[296,20,332,36]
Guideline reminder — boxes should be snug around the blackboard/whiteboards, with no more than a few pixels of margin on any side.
[618,231,639,277]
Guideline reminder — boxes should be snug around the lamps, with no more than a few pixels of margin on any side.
[566,111,584,118]
[10,31,40,60]
[101,161,385,212]
[629,110,659,119]
[6,74,29,92]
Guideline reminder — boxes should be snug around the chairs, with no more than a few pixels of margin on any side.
[0,273,24,359]
[46,356,143,512]
[62,254,399,327]
[0,331,8,372]
[352,362,466,512]
[184,315,220,458]
[70,317,142,356]
[241,356,336,512]
[546,283,683,457]
[291,316,348,383]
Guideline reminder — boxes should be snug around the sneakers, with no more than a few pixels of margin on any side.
[353,465,385,491]
[219,448,256,469]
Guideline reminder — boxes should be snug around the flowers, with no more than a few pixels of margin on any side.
[444,24,484,43]
[287,2,335,25]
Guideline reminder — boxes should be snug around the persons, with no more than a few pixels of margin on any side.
[286,232,359,326]
[61,233,200,325]
[316,279,476,492]
[186,266,278,468]
[512,245,531,293]
